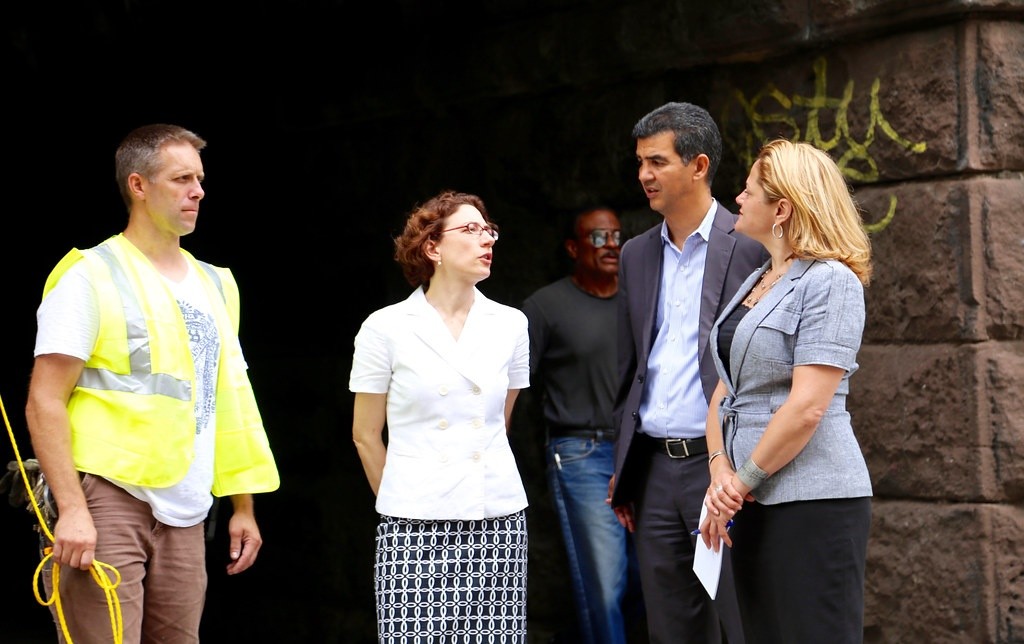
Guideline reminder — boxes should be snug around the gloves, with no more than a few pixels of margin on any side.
[0,458,42,505]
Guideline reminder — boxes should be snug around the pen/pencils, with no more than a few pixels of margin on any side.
[690,518,733,536]
[555,452,564,471]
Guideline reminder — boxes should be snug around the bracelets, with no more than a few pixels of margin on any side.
[736,456,770,489]
[708,450,729,467]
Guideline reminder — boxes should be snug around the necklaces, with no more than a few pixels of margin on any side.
[747,268,785,303]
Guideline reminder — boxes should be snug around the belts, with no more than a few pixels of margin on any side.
[646,437,708,459]
[555,429,615,440]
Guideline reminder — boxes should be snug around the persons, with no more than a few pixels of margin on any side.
[350,192,531,644]
[606,102,771,644]
[25,124,281,644]
[522,210,631,644]
[701,139,874,644]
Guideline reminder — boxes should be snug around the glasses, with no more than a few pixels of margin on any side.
[438,222,499,241]
[587,228,622,248]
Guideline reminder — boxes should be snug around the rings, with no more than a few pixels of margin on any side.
[714,486,723,492]
